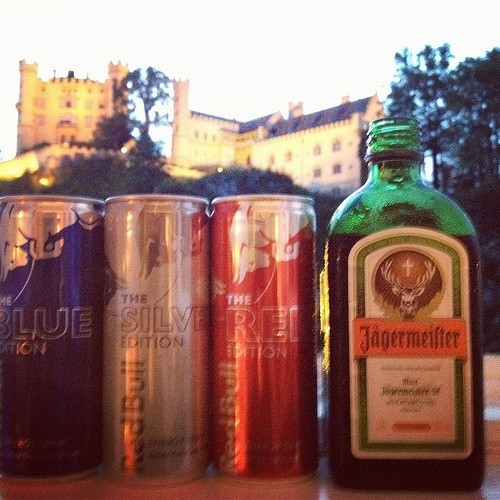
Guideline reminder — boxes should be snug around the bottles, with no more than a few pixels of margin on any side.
[320,115,488,491]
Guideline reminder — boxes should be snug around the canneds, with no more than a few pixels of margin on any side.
[0,194,104,484]
[210,194,320,488]
[104,194,210,487]
